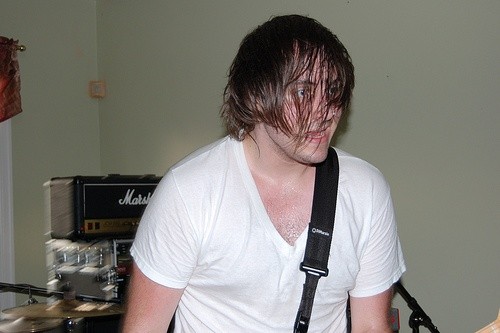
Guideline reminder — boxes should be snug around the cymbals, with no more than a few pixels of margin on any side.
[2,303,128,321]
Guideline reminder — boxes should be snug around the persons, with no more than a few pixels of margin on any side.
[120,12,407,333]
